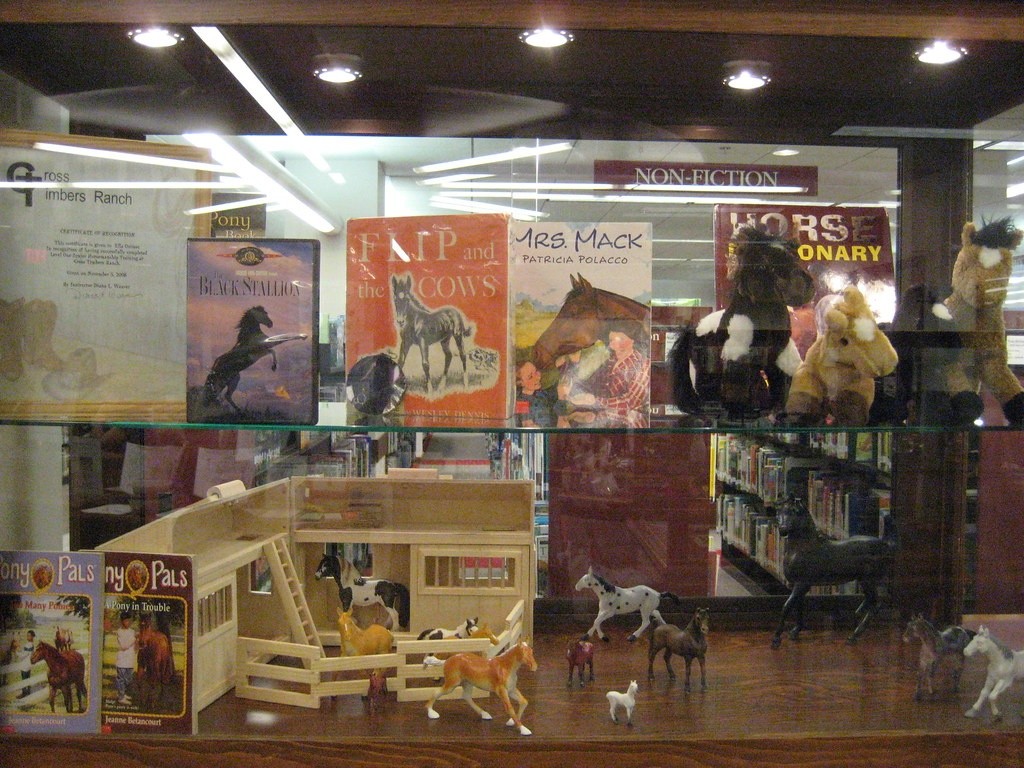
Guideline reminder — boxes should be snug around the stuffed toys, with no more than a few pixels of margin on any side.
[668,216,1023,428]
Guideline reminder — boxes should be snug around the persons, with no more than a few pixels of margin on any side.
[116,612,137,706]
[86,424,142,489]
[15,630,36,698]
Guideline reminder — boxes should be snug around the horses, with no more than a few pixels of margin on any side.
[642,606,713,696]
[562,637,642,729]
[199,304,310,416]
[900,609,1023,725]
[419,630,540,737]
[573,562,681,646]
[28,637,84,716]
[312,551,412,633]
[332,603,502,701]
[529,271,651,376]
[767,488,900,654]
[133,611,178,716]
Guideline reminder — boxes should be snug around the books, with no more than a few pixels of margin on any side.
[347,213,512,429]
[254,427,400,566]
[488,432,548,598]
[507,221,652,428]
[715,430,893,594]
[184,235,319,427]
[0,549,197,735]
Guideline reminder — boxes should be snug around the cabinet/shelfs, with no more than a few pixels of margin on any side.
[0,422,1024,768]
